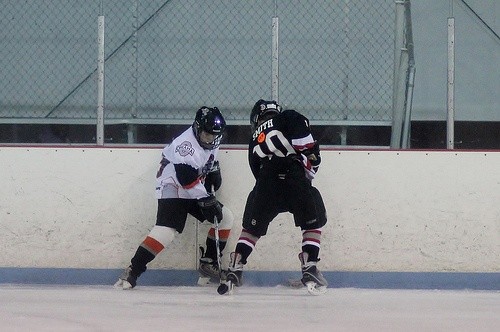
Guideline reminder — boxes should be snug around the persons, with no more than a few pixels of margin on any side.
[217,98,329,295]
[113,106,235,290]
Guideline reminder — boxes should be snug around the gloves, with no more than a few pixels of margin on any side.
[197,193,223,224]
[204,161,221,193]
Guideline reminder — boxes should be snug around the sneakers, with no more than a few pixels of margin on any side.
[224,251,244,295]
[298,250,328,295]
[114,265,138,289]
[197,245,229,287]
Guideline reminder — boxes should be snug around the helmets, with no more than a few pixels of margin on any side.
[250,98,282,133]
[192,106,226,150]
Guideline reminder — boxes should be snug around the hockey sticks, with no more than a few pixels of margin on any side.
[288,278,305,288]
[211,184,236,286]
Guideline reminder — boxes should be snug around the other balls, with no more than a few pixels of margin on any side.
[217,284,229,295]
